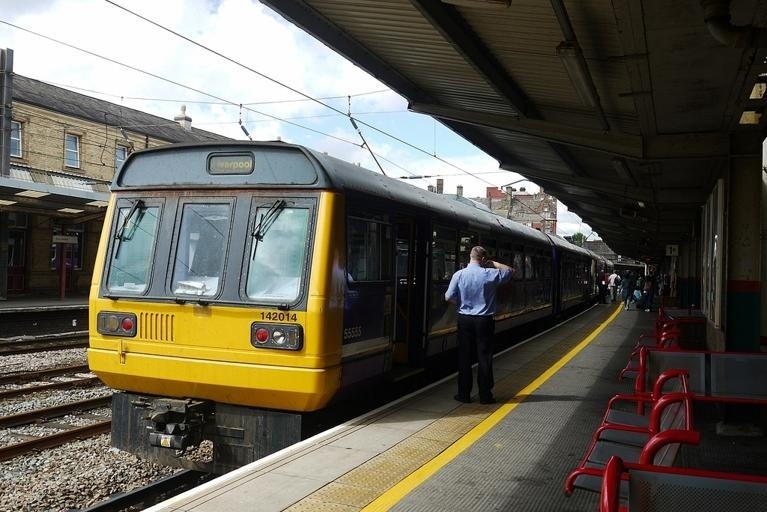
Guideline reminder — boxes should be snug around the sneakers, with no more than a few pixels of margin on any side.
[453,392,471,403]
[480,397,497,404]
[611,300,651,313]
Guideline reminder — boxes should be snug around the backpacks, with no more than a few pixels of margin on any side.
[635,292,641,301]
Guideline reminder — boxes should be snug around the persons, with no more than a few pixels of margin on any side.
[442,245,518,404]
[595,269,671,313]
[326,248,356,311]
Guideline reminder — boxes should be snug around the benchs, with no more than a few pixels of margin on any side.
[635,346,767,418]
[597,452,766,511]
[618,319,679,382]
[565,368,694,499]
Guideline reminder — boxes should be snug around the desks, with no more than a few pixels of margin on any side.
[658,306,706,323]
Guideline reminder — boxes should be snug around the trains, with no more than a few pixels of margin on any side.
[88,139,613,474]
